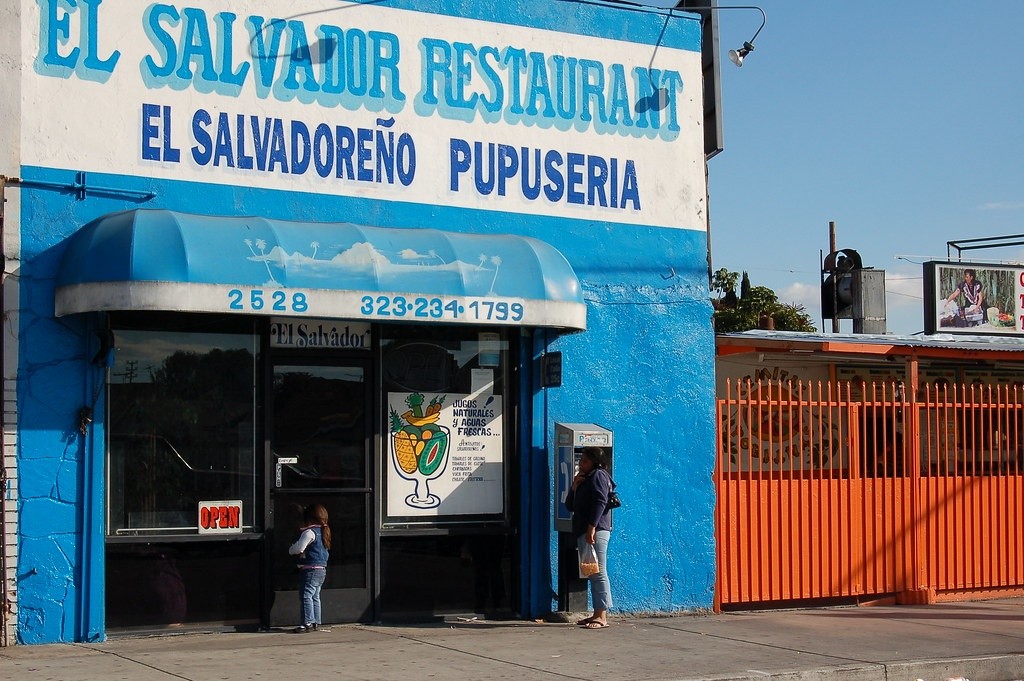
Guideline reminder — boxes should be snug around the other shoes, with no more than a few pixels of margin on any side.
[312,624,318,630]
[295,624,310,633]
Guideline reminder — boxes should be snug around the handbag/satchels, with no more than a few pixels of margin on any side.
[589,470,621,509]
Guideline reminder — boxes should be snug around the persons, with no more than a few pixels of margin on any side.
[566,447,614,629]
[287,506,331,634]
[944,268,989,327]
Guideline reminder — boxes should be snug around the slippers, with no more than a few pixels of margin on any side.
[588,621,609,628]
[577,619,589,625]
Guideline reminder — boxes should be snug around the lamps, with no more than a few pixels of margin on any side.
[91,345,116,369]
[659,6,766,67]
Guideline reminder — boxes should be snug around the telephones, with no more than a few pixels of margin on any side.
[579,460,584,475]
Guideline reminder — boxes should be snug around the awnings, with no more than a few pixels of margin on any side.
[55,209,587,335]
